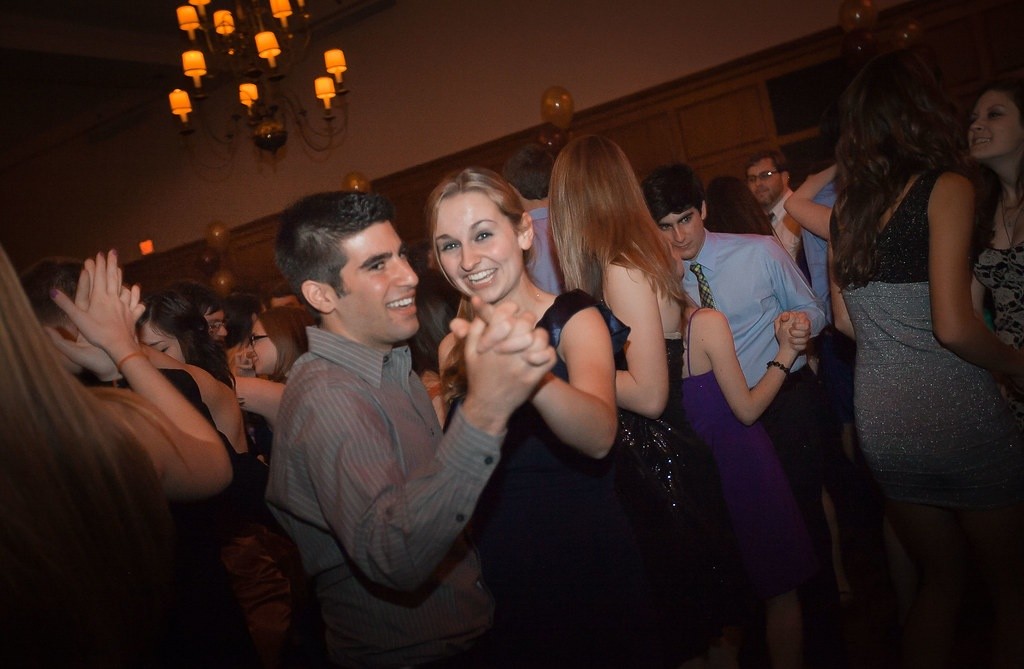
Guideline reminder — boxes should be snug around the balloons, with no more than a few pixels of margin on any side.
[839,0,877,36]
[209,266,235,301]
[541,85,576,130]
[341,171,369,193]
[196,248,219,277]
[206,220,230,254]
[537,122,569,158]
[888,17,922,48]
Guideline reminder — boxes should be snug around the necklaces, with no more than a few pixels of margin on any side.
[1000,186,1024,246]
[529,290,543,313]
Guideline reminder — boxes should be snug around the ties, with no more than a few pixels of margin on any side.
[690,260,717,311]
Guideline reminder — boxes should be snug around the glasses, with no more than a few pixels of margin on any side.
[207,319,228,336]
[744,170,778,183]
[249,333,268,346]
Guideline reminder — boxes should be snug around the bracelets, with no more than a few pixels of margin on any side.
[117,350,148,373]
[765,361,788,373]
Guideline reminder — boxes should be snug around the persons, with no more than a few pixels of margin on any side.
[0,48,1024,669]
